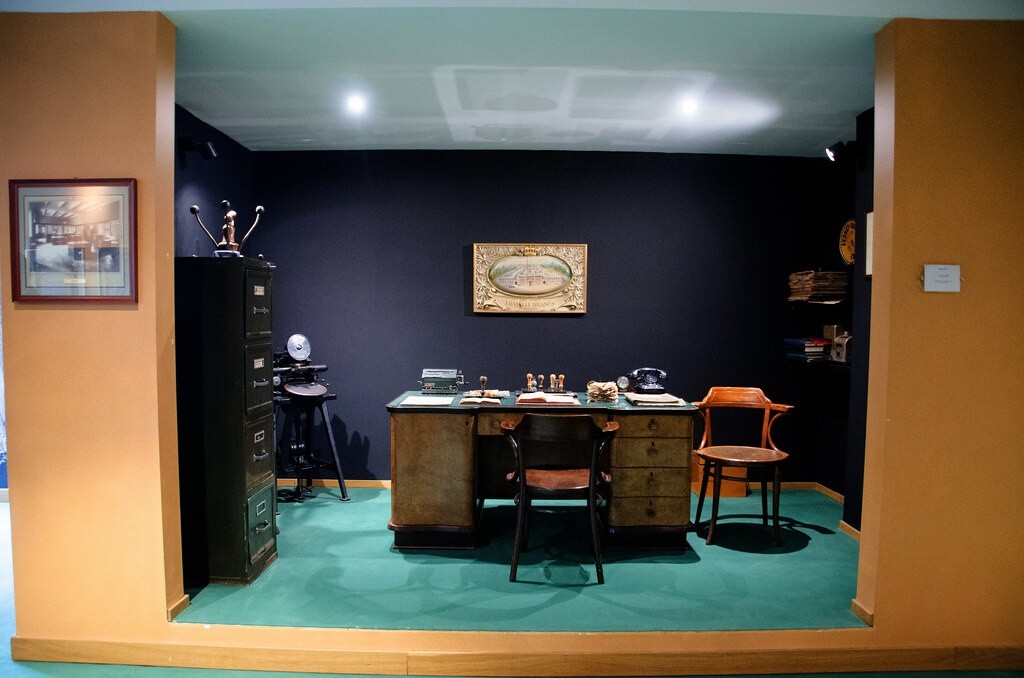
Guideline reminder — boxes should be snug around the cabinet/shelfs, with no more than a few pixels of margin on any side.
[174,256,279,587]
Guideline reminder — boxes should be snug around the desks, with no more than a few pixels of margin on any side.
[386,390,698,550]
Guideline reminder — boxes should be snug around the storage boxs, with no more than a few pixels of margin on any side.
[690,450,748,498]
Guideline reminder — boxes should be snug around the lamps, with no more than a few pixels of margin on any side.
[826,140,858,162]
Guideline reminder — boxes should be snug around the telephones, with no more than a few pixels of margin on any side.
[628,367,669,393]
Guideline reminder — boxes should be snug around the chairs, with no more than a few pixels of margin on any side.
[501,413,619,586]
[690,387,796,544]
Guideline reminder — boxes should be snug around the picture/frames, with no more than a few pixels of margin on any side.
[473,242,588,314]
[8,177,138,304]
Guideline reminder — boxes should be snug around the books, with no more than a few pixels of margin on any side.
[783,339,827,363]
[518,392,575,405]
[461,398,502,405]
